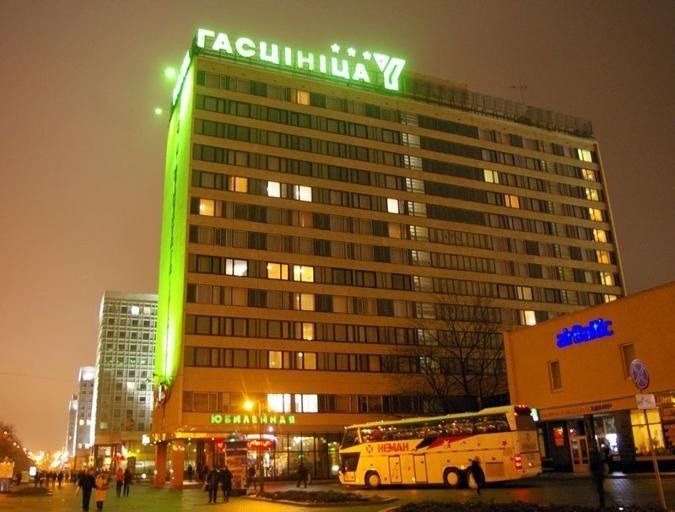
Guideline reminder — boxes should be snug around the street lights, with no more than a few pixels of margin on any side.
[245,399,267,496]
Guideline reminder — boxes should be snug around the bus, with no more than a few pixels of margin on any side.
[333,403,544,490]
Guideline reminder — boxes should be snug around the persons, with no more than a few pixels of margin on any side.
[295,462,311,488]
[469,454,485,494]
[13,461,256,512]
[600,437,616,475]
[587,437,608,508]
